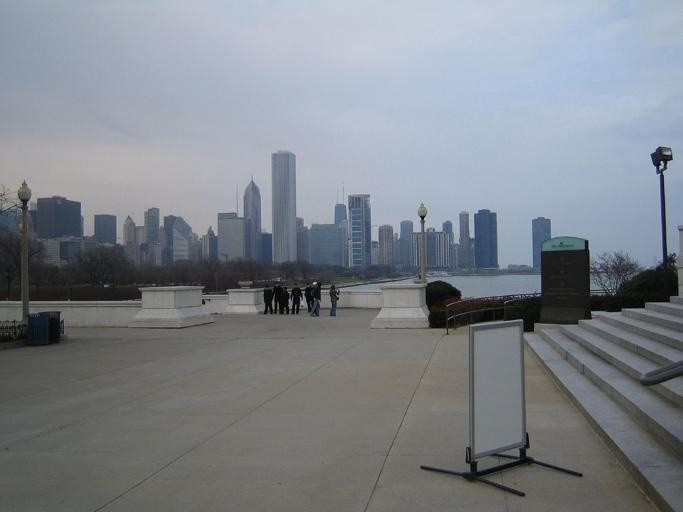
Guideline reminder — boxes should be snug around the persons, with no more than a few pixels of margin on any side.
[304,281,321,316]
[264,284,273,314]
[273,281,283,314]
[291,282,303,314]
[280,287,289,314]
[330,284,340,316]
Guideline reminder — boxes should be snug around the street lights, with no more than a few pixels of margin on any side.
[651,146,673,302]
[17,179,32,338]
[418,200,427,284]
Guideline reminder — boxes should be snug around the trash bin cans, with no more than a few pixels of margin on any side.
[26,311,50,346]
[45,311,62,344]
